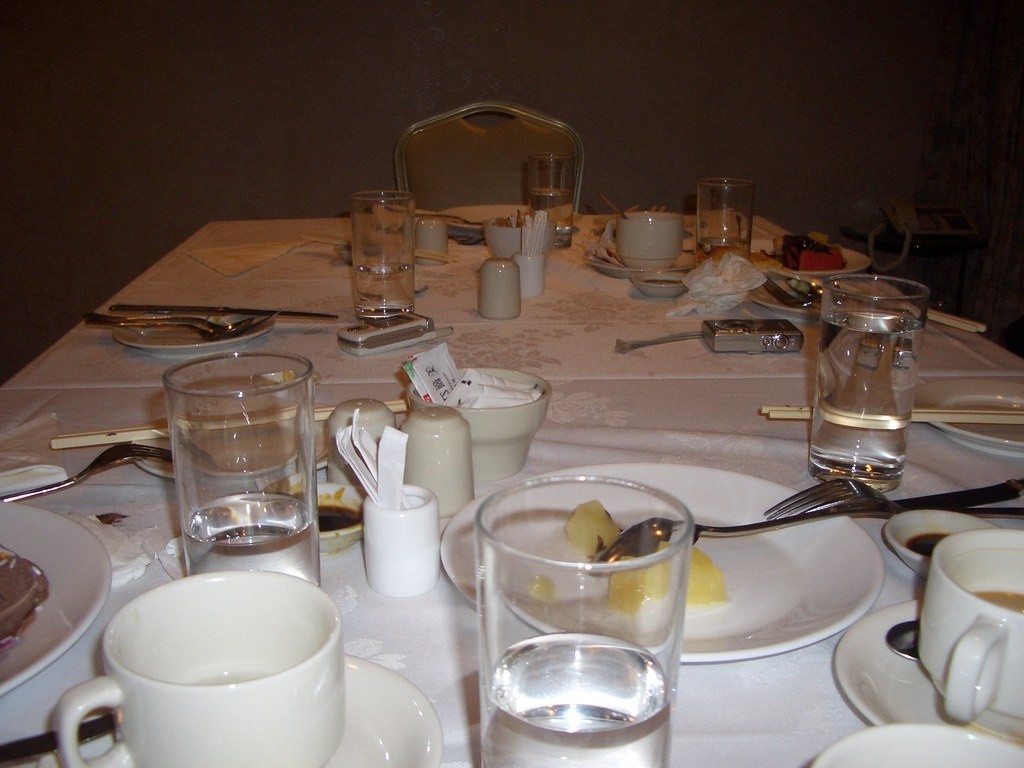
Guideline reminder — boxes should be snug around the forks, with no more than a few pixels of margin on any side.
[764,479,1024,523]
[0,441,173,502]
[760,275,822,307]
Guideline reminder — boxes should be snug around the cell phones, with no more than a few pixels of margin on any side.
[336,313,454,357]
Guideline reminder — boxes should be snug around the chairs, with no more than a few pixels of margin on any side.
[393,102,585,210]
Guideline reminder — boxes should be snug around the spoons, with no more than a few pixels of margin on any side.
[588,484,877,564]
[787,278,821,298]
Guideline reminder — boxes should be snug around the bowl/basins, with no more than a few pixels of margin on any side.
[407,368,551,480]
[289,483,363,556]
[680,267,767,310]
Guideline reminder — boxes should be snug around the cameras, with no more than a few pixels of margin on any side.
[701,319,804,355]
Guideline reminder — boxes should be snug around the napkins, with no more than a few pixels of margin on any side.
[187,235,318,278]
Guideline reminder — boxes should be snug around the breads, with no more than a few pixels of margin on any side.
[772,237,785,256]
[807,231,830,246]
[697,245,778,270]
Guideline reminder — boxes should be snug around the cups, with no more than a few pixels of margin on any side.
[616,212,684,268]
[58,152,1024,768]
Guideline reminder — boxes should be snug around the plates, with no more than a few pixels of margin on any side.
[929,395,1023,458]
[0,501,112,693]
[582,251,696,278]
[629,270,689,297]
[748,276,903,319]
[112,323,273,358]
[326,654,444,768]
[181,441,300,476]
[131,441,326,480]
[884,510,1002,581]
[755,247,872,280]
[441,463,884,664]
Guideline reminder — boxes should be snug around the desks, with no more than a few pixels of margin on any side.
[1,207,1024,768]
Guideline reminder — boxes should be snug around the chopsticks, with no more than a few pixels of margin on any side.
[301,234,458,263]
[50,399,407,450]
[760,404,1024,425]
